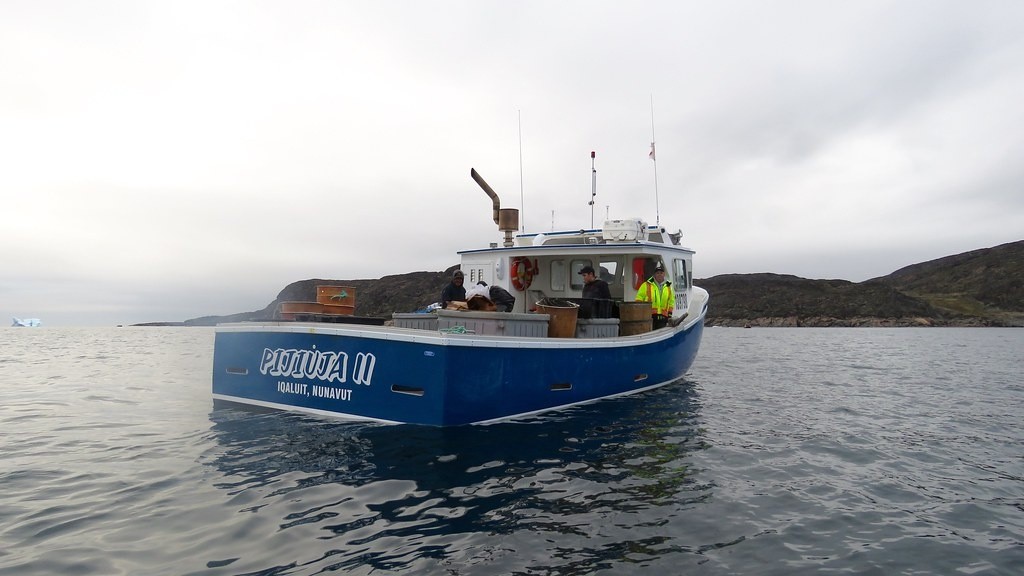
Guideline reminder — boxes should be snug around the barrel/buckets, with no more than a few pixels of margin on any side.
[535,301,578,338]
[279,301,324,321]
[317,285,356,314]
[619,302,652,336]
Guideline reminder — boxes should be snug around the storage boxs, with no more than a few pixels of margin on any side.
[550,297,615,318]
[436,309,551,338]
[392,312,438,331]
[295,313,385,325]
[576,318,620,337]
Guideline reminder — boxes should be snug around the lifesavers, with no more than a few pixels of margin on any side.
[510,256,534,292]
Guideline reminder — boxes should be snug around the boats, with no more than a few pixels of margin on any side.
[213,94,709,430]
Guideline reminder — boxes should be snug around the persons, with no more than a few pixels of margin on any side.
[634,262,675,331]
[441,269,516,313]
[577,266,612,300]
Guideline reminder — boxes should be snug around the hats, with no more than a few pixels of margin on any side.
[578,266,594,274]
[454,272,463,279]
[655,260,665,272]
